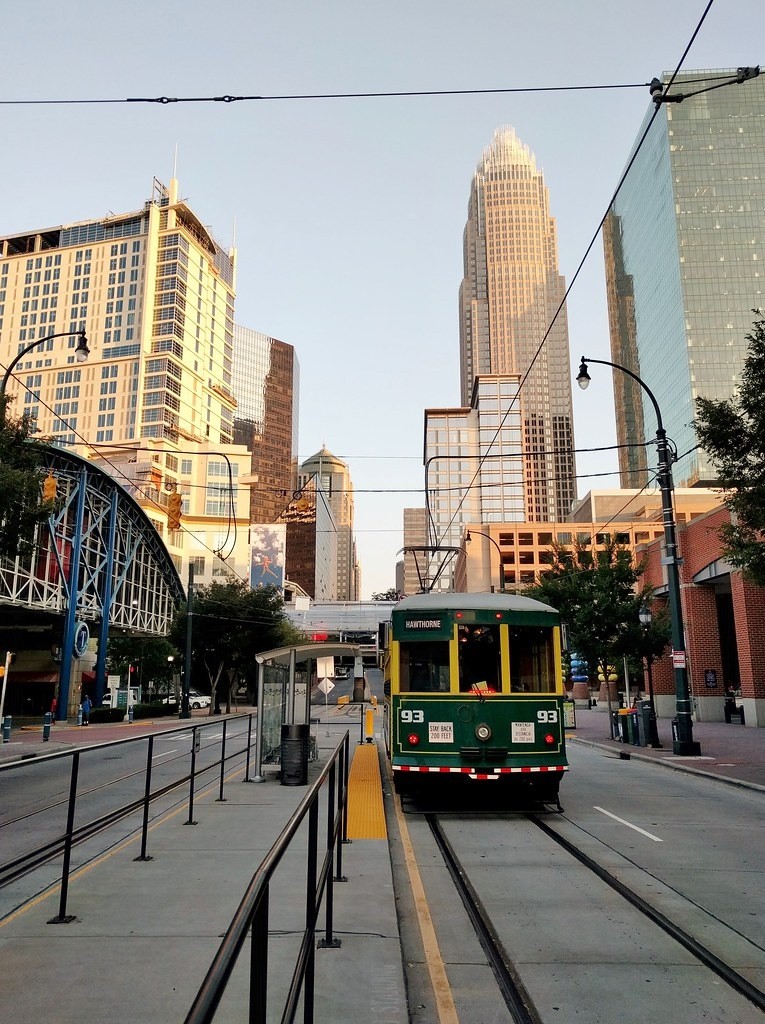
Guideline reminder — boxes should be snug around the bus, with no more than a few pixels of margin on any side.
[378,594,570,814]
[335,666,350,680]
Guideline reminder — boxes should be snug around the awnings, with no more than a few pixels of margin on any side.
[5,671,59,683]
[83,671,107,681]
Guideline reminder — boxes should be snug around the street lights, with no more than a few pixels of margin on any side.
[0,330,90,436]
[464,529,506,594]
[576,357,703,757]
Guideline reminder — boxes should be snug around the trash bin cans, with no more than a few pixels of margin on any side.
[279,723,310,786]
[562,697,576,729]
[636,700,660,747]
[611,710,640,746]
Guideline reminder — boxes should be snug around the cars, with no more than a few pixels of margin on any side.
[102,690,211,709]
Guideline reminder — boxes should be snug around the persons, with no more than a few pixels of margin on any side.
[82,695,92,726]
[726,685,735,697]
[51,697,57,725]
[631,691,645,708]
